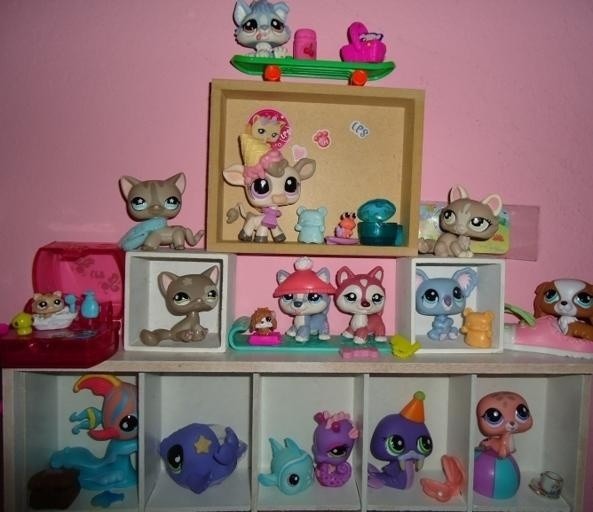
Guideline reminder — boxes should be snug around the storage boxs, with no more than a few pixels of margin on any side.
[0,240,126,370]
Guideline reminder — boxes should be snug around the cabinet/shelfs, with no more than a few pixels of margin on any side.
[0,66,592,512]
